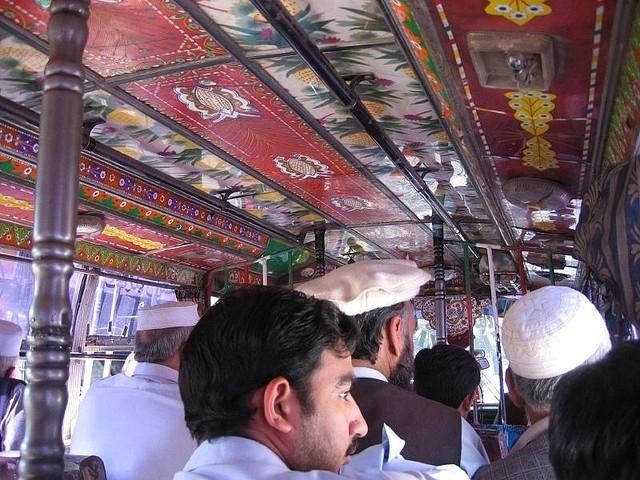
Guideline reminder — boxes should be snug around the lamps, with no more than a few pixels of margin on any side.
[506,52,539,92]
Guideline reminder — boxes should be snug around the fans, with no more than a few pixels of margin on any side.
[502,175,573,210]
[75,212,106,238]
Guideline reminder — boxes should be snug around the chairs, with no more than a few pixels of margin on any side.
[474,428,508,463]
[0,450,107,480]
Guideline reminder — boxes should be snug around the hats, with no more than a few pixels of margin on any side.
[293,259,431,316]
[0,320,24,358]
[501,286,612,379]
[135,301,201,331]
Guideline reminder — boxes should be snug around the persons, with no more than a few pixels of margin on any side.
[68,301,201,480]
[177,283,367,478]
[474,287,640,480]
[292,260,491,478]
[0,319,26,451]
